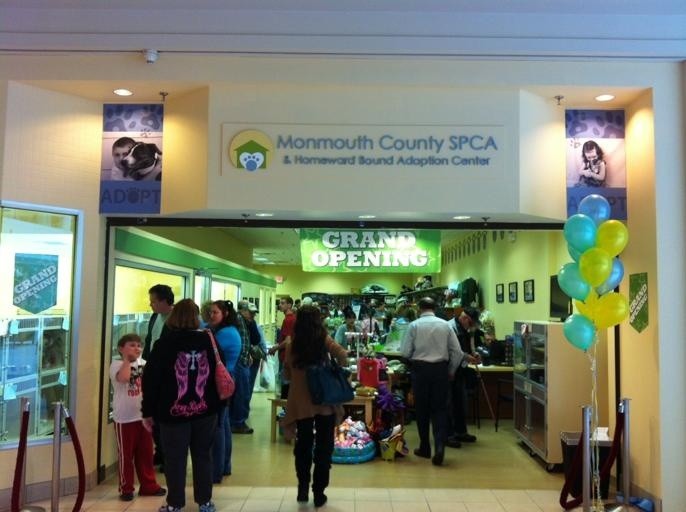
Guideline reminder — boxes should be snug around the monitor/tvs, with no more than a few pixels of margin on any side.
[550,275,574,323]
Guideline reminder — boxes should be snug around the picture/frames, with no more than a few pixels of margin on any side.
[496,284,503,302]
[524,280,534,301]
[509,282,517,302]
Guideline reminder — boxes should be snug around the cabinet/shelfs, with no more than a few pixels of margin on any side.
[514,321,608,474]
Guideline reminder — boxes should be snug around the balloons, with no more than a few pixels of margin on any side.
[557,193,628,349]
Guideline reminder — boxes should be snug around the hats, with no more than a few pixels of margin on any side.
[241,302,259,313]
[464,307,481,325]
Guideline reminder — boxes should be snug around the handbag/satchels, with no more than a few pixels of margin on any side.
[215,361,235,400]
[252,347,263,359]
[306,363,353,404]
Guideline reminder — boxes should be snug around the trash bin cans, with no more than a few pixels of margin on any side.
[560,427,614,500]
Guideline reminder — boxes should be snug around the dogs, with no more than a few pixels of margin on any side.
[120,141,162,181]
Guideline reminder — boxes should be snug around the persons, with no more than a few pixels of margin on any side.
[578,141,607,186]
[283,305,347,506]
[110,136,161,181]
[140,298,227,511]
[109,284,495,502]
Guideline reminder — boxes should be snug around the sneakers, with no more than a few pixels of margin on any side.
[414,448,430,458]
[138,488,166,496]
[158,502,216,512]
[231,423,253,433]
[121,492,133,500]
[446,434,476,448]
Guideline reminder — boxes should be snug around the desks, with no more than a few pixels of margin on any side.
[466,358,544,431]
[267,393,375,445]
